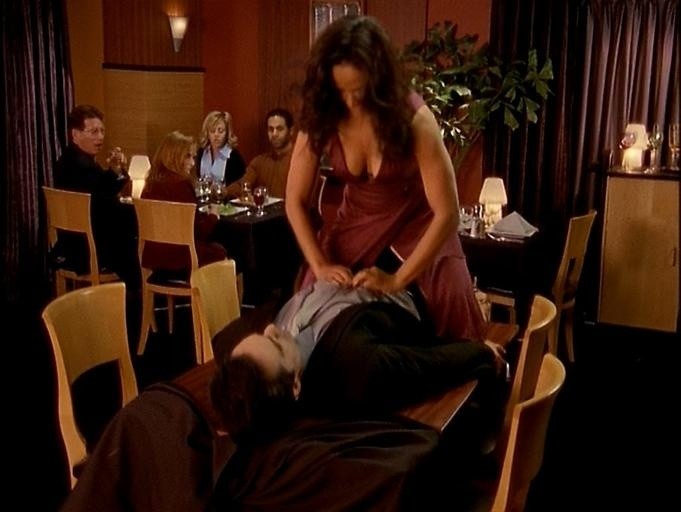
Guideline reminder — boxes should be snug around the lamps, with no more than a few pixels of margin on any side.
[168,15,190,53]
[127,155,152,196]
[479,177,509,222]
[623,124,650,170]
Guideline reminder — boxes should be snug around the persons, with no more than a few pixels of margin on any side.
[225,108,295,304]
[139,131,226,284]
[284,14,487,345]
[192,111,244,187]
[47,104,141,292]
[209,264,509,431]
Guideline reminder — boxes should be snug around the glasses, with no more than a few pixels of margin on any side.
[79,127,105,135]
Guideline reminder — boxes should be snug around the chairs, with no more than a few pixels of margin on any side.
[493,355,566,512]
[489,209,598,364]
[132,196,243,355]
[487,295,556,437]
[42,185,120,298]
[42,283,139,491]
[189,260,243,365]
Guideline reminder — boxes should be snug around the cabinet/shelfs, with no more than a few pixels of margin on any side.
[598,169,681,335]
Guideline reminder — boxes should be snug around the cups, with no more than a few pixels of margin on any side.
[460,204,474,235]
[240,181,254,207]
[254,187,267,217]
[196,172,229,204]
[644,123,664,174]
[617,130,637,173]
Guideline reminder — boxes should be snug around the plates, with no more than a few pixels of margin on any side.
[229,196,284,208]
[199,203,250,216]
[485,226,539,238]
